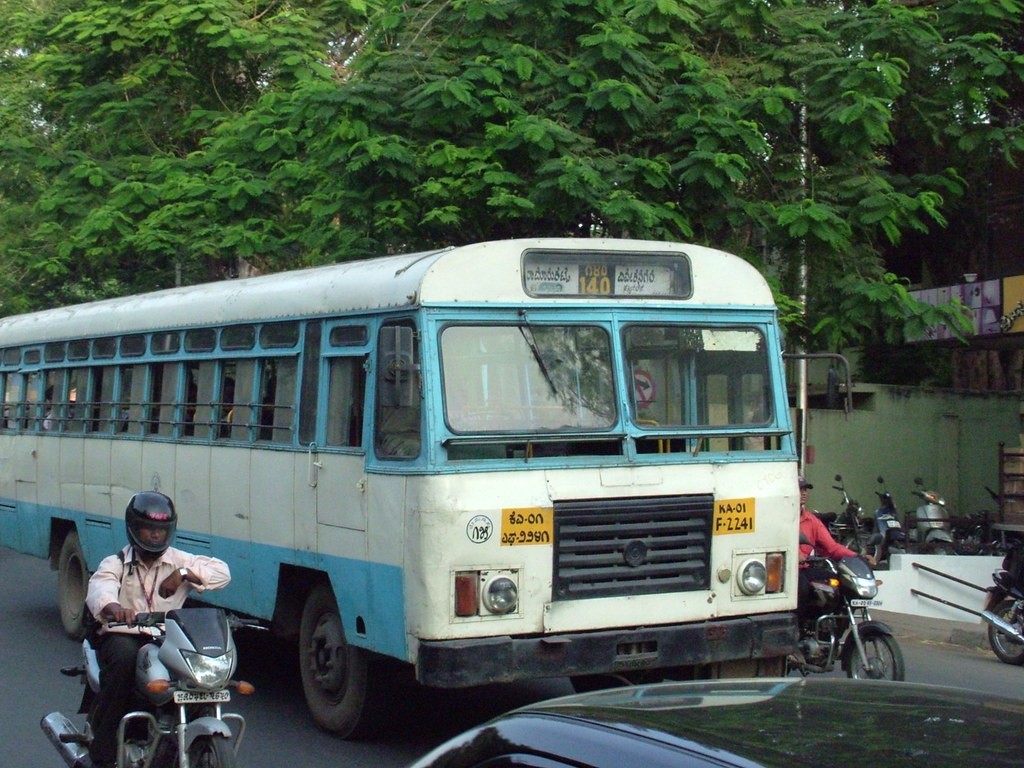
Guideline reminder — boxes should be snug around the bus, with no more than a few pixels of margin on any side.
[1,237,856,745]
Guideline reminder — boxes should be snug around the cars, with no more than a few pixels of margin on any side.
[411,680,1024,767]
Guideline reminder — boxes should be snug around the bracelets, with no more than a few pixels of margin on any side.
[177,567,188,581]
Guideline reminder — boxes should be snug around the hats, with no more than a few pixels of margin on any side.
[798,475,814,489]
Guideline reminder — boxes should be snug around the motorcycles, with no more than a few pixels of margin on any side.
[38,606,269,768]
[798,531,906,682]
[981,572,1024,662]
[816,475,1020,567]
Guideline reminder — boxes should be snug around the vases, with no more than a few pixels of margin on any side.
[964,273,978,283]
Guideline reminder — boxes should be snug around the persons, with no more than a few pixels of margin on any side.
[85,491,232,768]
[797,474,877,614]
[43,376,276,430]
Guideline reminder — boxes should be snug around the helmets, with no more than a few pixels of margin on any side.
[125,492,177,558]
